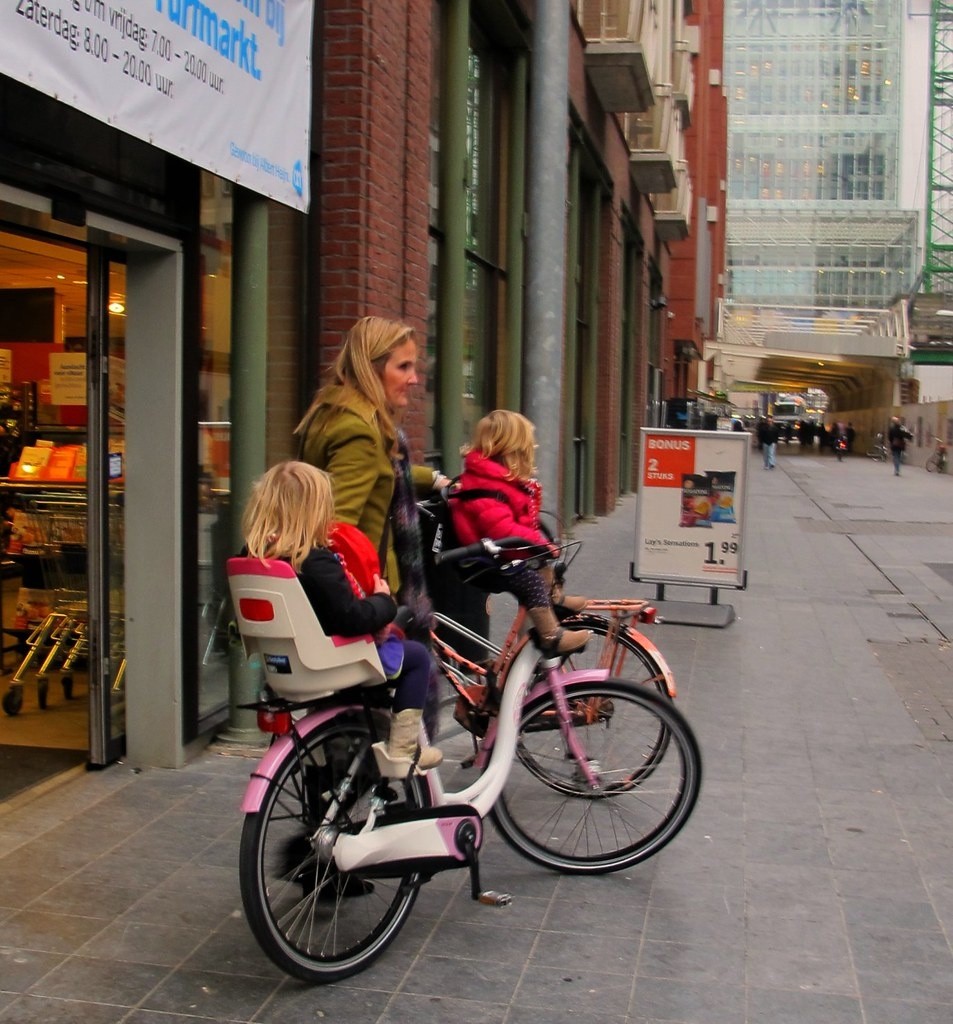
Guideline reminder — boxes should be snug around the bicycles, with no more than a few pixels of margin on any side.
[238,505,711,987]
[423,562,681,795]
[867,432,888,460]
[926,437,949,475]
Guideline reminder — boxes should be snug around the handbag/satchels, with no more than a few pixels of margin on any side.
[891,437,906,450]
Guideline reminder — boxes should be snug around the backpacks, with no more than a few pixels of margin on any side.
[416,474,519,611]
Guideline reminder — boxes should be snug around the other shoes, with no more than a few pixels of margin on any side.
[303,858,374,900]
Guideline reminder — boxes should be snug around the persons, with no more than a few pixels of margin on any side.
[888,416,912,477]
[757,416,780,470]
[235,459,442,772]
[270,315,455,903]
[779,419,856,454]
[732,420,744,432]
[444,403,594,657]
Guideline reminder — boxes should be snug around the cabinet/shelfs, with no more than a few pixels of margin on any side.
[0,433,91,657]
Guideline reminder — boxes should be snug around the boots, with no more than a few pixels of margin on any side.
[527,607,591,655]
[387,707,443,770]
[536,565,588,612]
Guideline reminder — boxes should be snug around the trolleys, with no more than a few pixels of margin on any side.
[0,492,235,717]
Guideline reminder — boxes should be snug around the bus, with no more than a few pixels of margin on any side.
[772,402,808,438]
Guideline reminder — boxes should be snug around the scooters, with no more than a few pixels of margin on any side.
[835,435,847,461]
